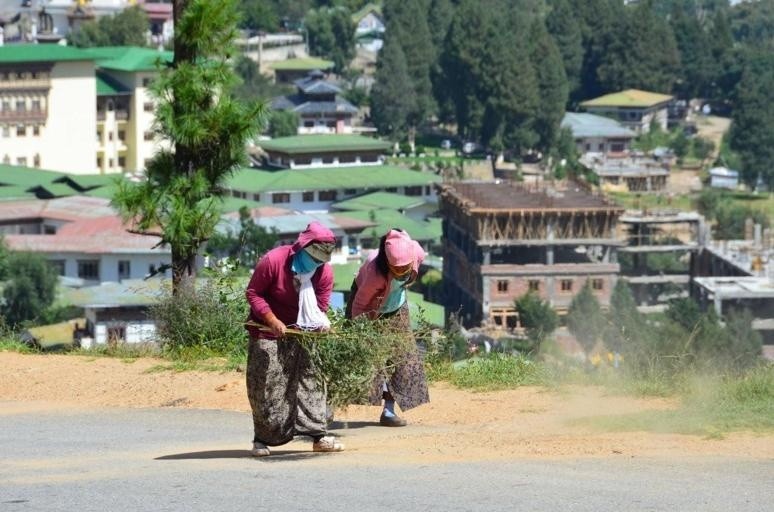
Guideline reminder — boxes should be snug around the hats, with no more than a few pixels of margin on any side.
[305,242,333,265]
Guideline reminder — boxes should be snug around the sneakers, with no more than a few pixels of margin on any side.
[380,412,407,427]
[314,437,345,453]
[252,445,270,456]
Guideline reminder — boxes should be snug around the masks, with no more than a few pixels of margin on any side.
[389,268,412,283]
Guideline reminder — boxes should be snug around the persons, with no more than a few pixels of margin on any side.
[336,229,427,434]
[245,219,346,454]
